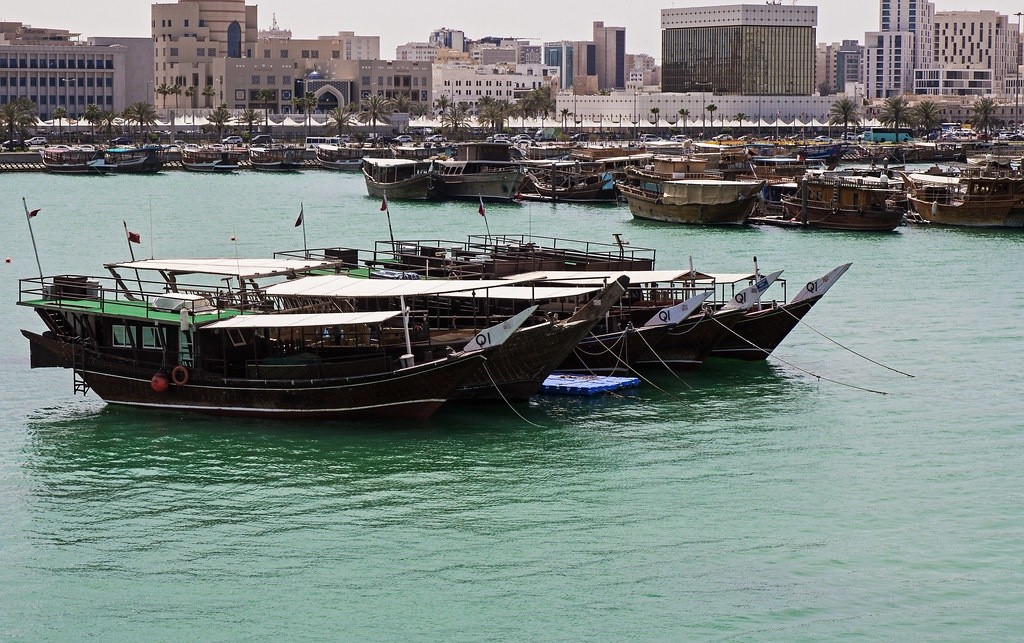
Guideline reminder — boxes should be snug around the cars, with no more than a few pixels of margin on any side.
[671,134,692,143]
[394,134,413,142]
[840,131,858,141]
[814,134,834,142]
[510,134,532,142]
[640,133,662,143]
[424,134,448,143]
[1,139,31,149]
[106,136,132,145]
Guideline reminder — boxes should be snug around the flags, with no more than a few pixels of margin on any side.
[295,212,302,228]
[129,232,140,244]
[29,209,41,218]
[479,203,484,216]
[380,198,386,211]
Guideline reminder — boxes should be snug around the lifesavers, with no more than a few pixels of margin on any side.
[172,366,190,385]
[832,207,839,215]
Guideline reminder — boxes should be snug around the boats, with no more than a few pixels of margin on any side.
[0,125,1024,428]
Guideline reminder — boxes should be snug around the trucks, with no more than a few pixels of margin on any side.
[533,130,556,141]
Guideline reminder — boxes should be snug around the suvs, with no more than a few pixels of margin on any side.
[568,132,590,142]
[486,134,512,142]
[220,135,244,144]
[249,134,273,144]
[24,136,48,145]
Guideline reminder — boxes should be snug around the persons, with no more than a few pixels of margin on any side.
[870,159,875,170]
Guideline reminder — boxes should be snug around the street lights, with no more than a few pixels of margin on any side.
[62,78,76,147]
[146,80,154,125]
[853,81,859,142]
[633,89,639,147]
[215,79,222,125]
[758,84,768,142]
[574,82,582,135]
[297,80,311,137]
[695,82,713,140]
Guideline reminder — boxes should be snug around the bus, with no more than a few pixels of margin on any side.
[304,136,343,151]
[863,126,916,143]
